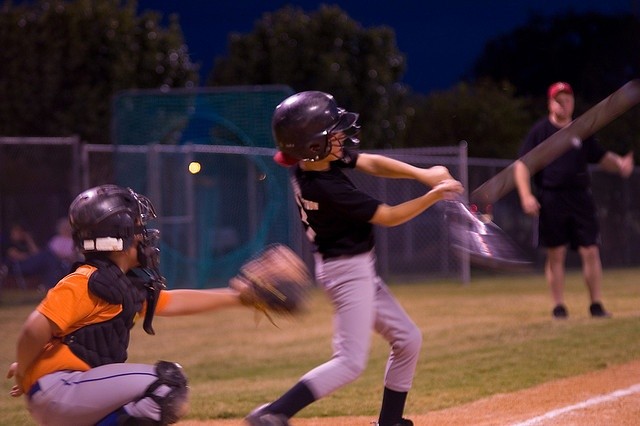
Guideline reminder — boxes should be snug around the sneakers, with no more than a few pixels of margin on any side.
[588,301,609,316]
[552,304,569,318]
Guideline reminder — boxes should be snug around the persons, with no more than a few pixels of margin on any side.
[244,89,465,426]
[1,221,39,291]
[513,80,634,319]
[35,215,86,295]
[7,185,309,426]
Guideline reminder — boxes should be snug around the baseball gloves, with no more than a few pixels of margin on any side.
[227,243,315,331]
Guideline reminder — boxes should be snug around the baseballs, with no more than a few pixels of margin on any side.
[190,161,202,173]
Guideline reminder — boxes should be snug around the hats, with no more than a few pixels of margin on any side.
[547,82,573,99]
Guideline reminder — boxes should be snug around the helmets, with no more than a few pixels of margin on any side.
[69,184,159,274]
[273,91,360,166]
